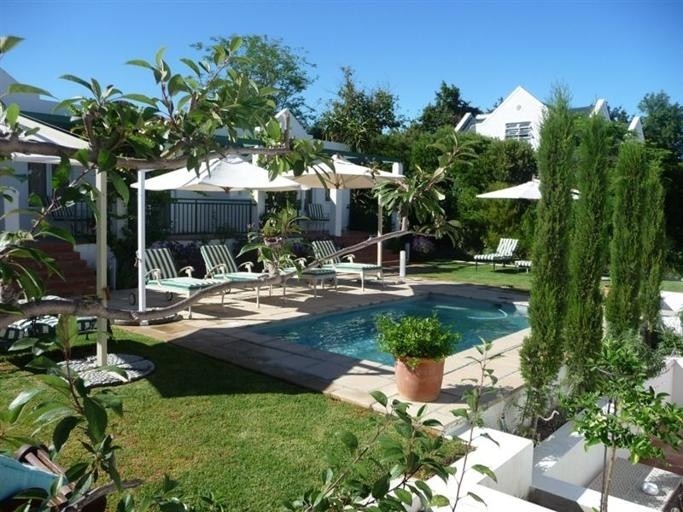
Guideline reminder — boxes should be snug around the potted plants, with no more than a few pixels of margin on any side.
[374,307,462,403]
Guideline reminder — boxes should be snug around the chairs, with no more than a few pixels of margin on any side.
[127,240,382,319]
[472,238,520,272]
[306,203,329,231]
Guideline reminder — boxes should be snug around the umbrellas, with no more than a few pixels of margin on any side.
[134,147,311,198]
[279,153,405,194]
[472,174,581,203]
[1,107,110,168]
